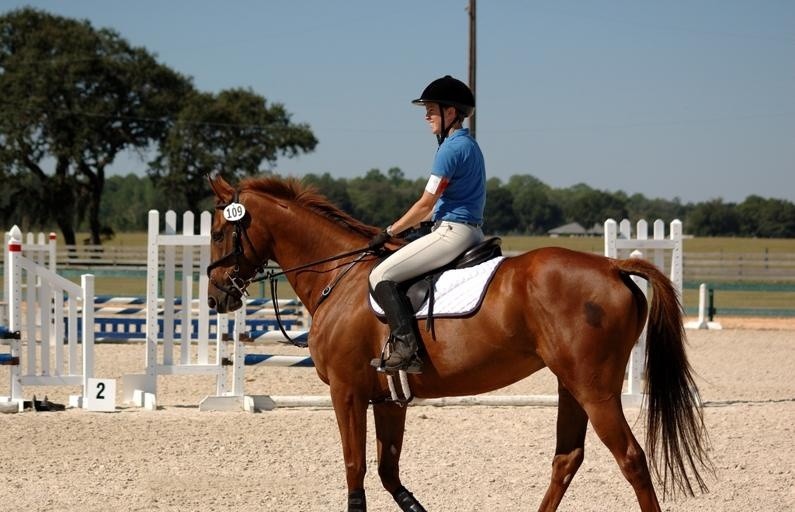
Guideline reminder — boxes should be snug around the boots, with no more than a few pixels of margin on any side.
[370,280,424,375]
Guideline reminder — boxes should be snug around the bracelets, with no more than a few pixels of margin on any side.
[387,225,396,238]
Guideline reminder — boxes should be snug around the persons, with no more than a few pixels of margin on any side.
[369,76,486,372]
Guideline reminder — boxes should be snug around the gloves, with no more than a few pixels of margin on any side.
[396,226,415,238]
[369,225,393,250]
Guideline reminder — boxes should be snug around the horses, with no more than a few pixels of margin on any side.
[207,171,718,512]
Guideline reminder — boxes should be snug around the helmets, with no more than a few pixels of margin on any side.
[411,75,475,108]
